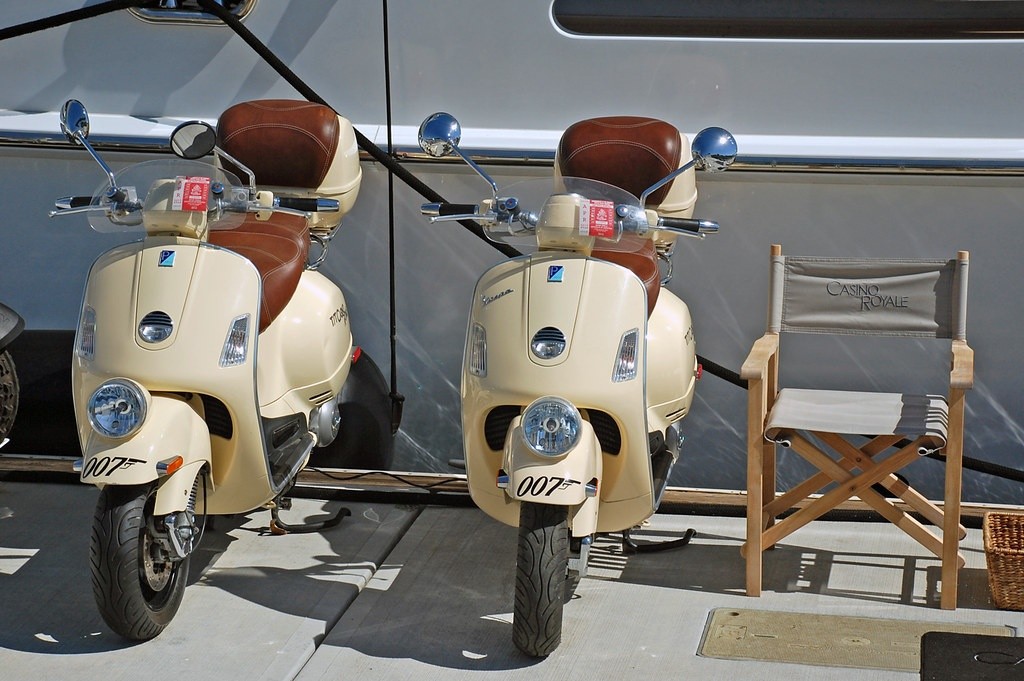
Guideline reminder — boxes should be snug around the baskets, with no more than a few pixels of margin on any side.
[983,511,1024,612]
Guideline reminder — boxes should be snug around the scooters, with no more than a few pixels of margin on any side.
[59,96,360,643]
[0,305,25,448]
[412,111,739,655]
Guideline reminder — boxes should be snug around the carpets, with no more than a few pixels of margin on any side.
[696,607,1024,681]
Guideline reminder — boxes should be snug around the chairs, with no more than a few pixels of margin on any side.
[741,245,973,610]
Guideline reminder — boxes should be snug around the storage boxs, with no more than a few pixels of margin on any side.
[554,117,698,247]
[213,100,362,229]
